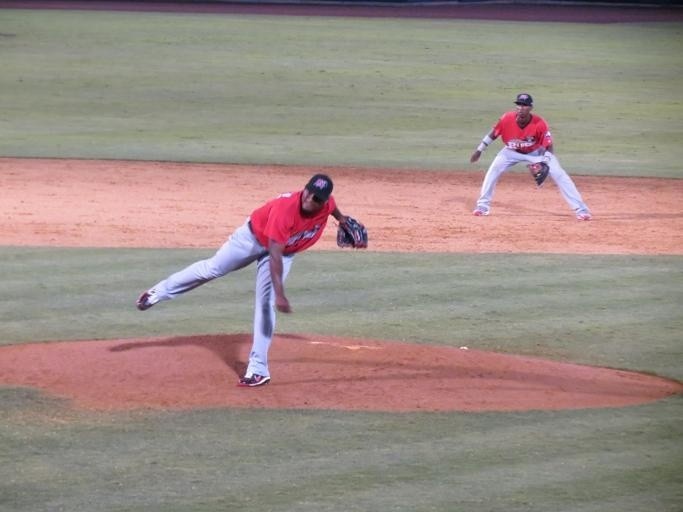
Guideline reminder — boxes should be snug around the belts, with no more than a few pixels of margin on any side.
[247,217,264,248]
[515,149,533,155]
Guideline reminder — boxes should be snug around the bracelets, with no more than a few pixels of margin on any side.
[482,134,493,145]
[543,151,553,160]
[476,143,487,152]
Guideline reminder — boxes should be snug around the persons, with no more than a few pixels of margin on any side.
[133,172,368,388]
[469,93,595,222]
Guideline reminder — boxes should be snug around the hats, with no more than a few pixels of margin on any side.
[305,173,333,199]
[514,92,535,107]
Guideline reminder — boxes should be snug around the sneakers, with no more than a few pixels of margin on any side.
[576,212,591,221]
[238,372,271,386]
[136,288,153,310]
[472,208,491,216]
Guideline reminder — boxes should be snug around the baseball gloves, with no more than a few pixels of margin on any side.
[527,162,549,185]
[337,216,368,248]
